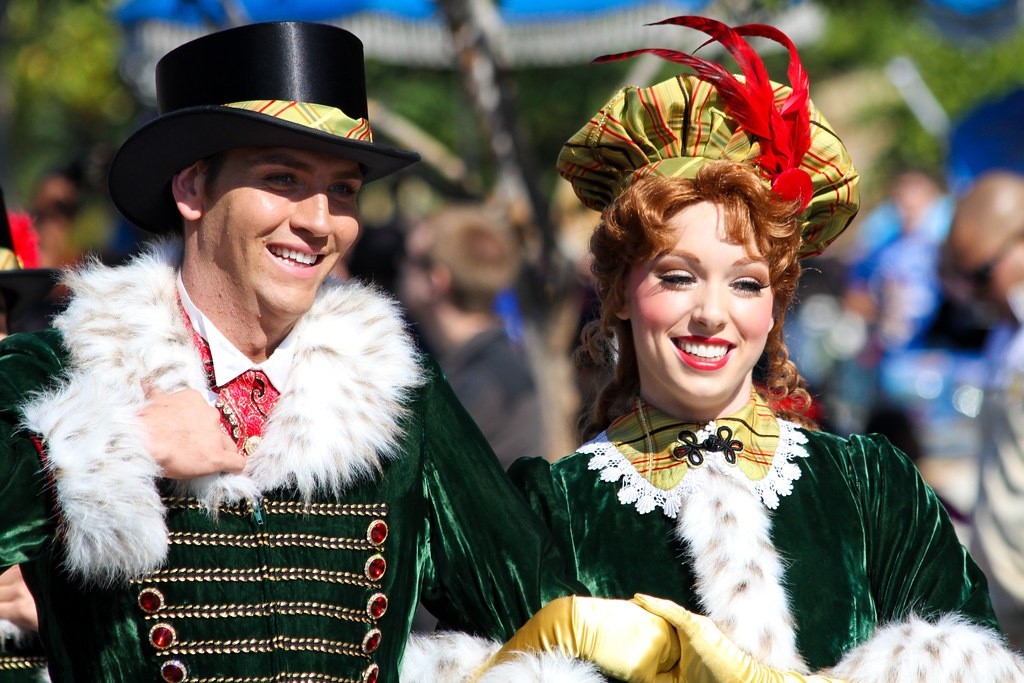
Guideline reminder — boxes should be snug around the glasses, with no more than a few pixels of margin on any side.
[943,233,1024,300]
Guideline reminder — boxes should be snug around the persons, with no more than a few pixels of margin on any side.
[1,170,1024,649]
[436,73,1024,683]
[0,22,543,683]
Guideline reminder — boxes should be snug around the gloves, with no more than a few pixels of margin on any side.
[630,593,851,683]
[466,595,681,683]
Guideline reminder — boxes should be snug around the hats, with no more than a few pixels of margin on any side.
[555,15,859,260]
[943,82,1024,190]
[108,19,420,240]
